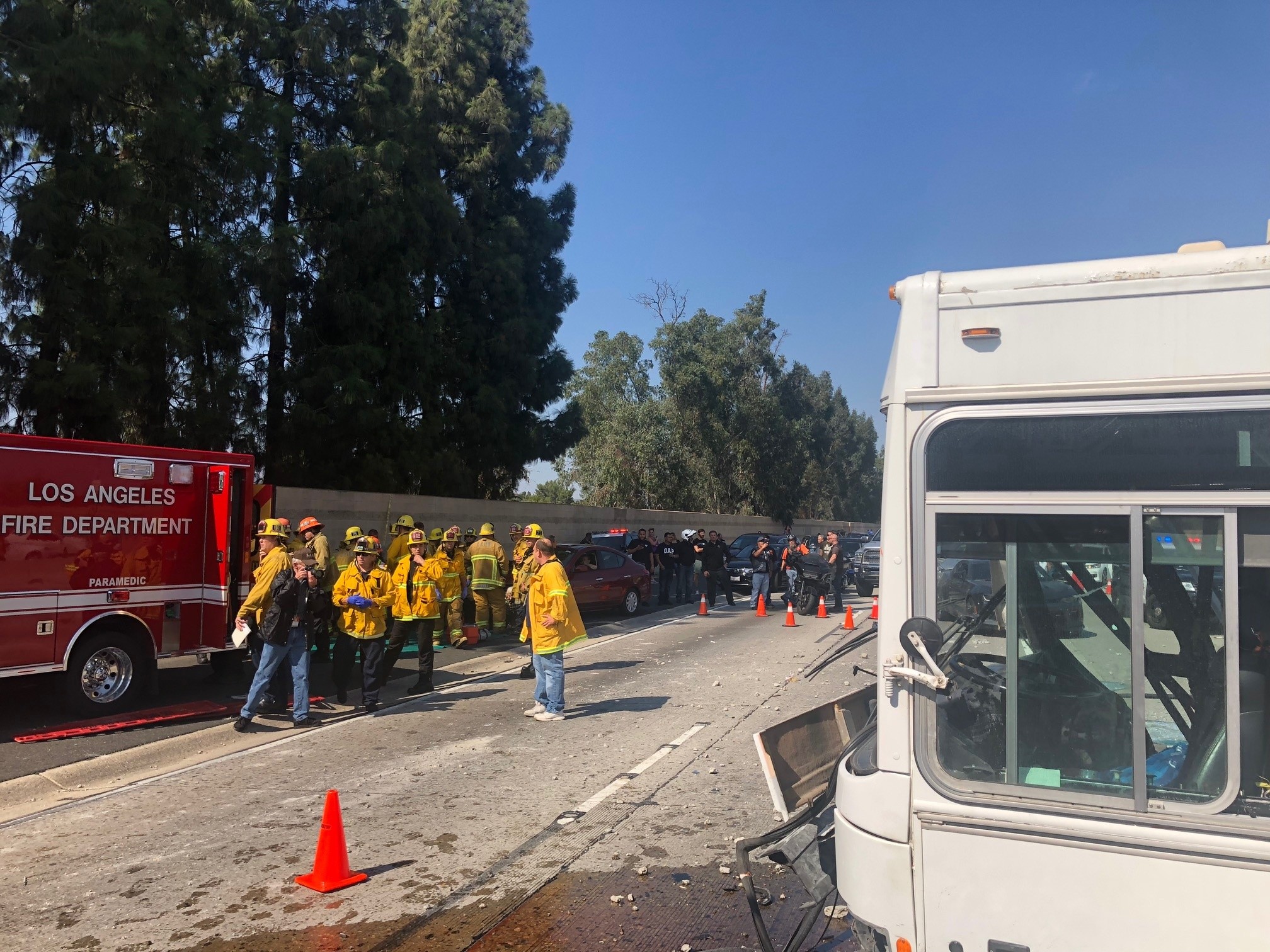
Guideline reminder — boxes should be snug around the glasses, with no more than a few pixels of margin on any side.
[828,538,835,540]
[827,534,832,536]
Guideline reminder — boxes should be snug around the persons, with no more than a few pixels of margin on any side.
[429,528,444,556]
[524,538,588,721]
[297,516,340,673]
[260,518,292,568]
[463,523,510,638]
[332,536,397,713]
[464,527,477,624]
[380,529,441,695]
[519,524,544,680]
[435,526,466,555]
[582,528,845,614]
[414,521,424,531]
[234,517,288,715]
[384,523,398,564]
[433,531,468,649]
[489,523,500,544]
[336,526,364,574]
[232,549,322,733]
[367,530,382,561]
[508,522,524,636]
[386,514,415,646]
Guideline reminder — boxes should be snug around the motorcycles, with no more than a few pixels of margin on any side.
[786,552,832,615]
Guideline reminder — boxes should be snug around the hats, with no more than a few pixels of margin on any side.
[757,537,765,542]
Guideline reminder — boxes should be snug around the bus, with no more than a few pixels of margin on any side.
[733,238,1270,951]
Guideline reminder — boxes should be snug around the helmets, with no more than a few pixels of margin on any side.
[254,516,325,538]
[787,534,797,541]
[429,522,543,541]
[344,515,431,554]
[681,529,696,541]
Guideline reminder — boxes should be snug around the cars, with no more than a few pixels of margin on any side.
[506,544,652,620]
[1111,564,1246,630]
[722,531,873,595]
[590,529,648,562]
[937,559,1083,635]
[1060,562,1114,584]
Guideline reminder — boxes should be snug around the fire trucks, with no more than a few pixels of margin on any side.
[0,433,278,721]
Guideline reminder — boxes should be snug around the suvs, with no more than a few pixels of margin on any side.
[854,528,881,596]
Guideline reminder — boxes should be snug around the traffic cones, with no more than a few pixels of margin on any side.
[293,791,369,893]
[696,593,710,616]
[1104,579,1112,595]
[815,595,831,618]
[868,597,878,619]
[755,593,769,617]
[782,602,799,627]
[840,605,857,631]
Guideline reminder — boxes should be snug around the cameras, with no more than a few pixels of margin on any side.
[304,566,324,579]
[766,544,771,548]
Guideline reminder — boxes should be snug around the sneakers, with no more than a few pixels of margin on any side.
[523,700,566,722]
[650,579,659,585]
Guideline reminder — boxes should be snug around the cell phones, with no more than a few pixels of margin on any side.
[640,544,643,547]
[707,574,711,578]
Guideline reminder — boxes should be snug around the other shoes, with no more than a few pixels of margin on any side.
[234,680,434,729]
[432,624,510,650]
[657,594,844,613]
